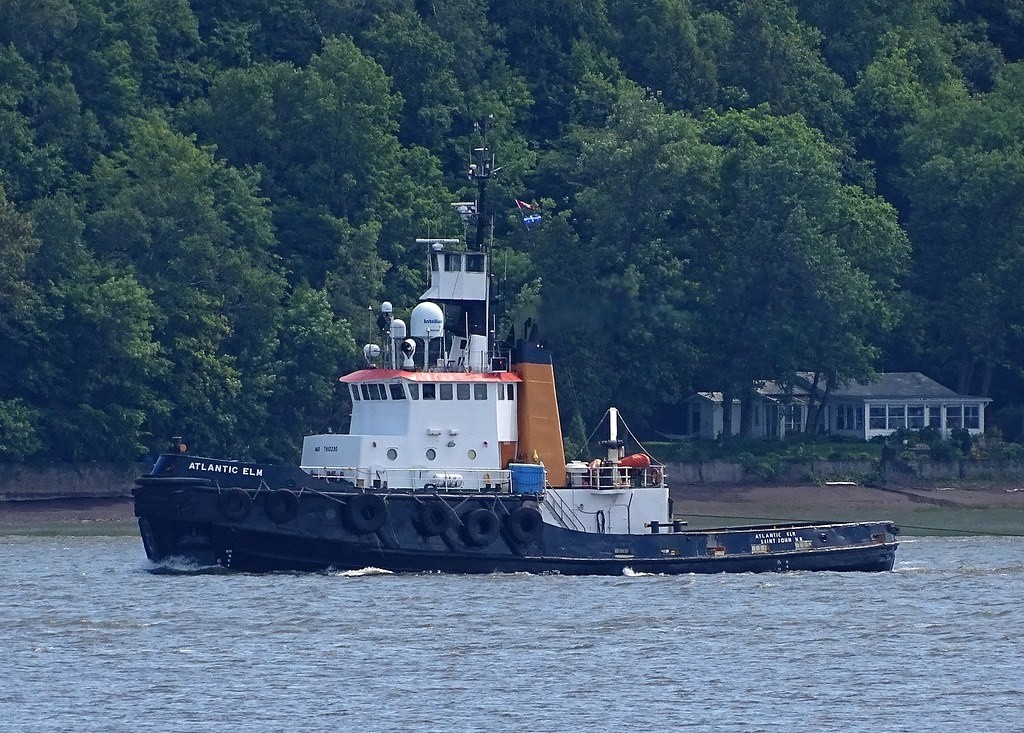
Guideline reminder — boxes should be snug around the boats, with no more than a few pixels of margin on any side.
[130,114,904,574]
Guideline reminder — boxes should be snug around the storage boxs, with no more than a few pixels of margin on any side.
[509,463,545,495]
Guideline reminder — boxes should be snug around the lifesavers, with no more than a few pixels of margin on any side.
[462,508,500,547]
[651,468,660,486]
[265,489,300,523]
[507,506,545,545]
[413,501,451,537]
[221,488,254,520]
[343,493,388,533]
